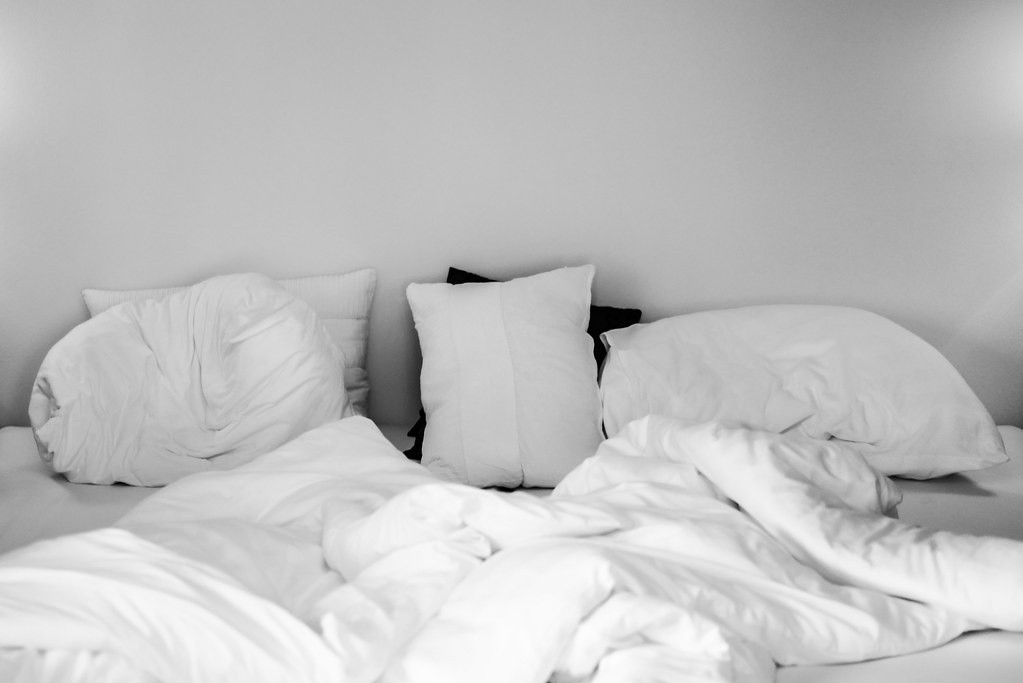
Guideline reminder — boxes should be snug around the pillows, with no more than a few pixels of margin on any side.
[27,271,354,489]
[81,267,377,419]
[406,265,642,438]
[404,265,607,489]
[599,303,1009,481]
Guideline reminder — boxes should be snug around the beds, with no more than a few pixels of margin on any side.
[0,423,1023,683]
